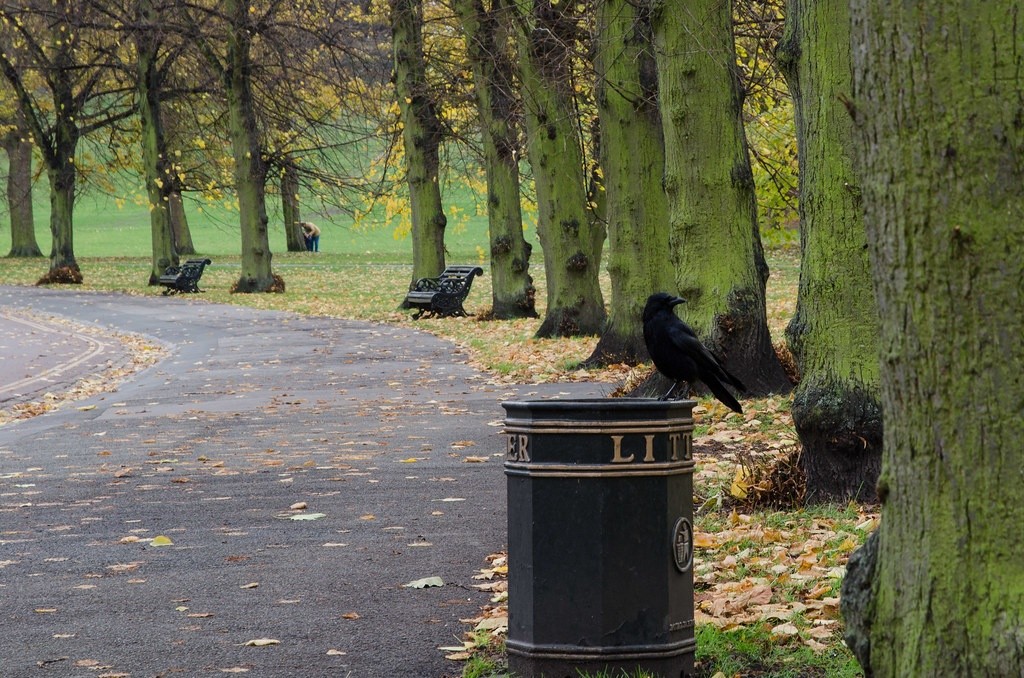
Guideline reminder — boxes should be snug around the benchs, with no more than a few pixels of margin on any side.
[405,264,484,320]
[158,258,211,294]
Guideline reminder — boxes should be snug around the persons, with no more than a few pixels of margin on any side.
[300,221,321,252]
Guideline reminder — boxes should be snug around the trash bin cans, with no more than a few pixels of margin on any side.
[501,398,698,678]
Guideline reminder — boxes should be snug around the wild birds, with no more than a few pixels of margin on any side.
[643,293,747,414]
